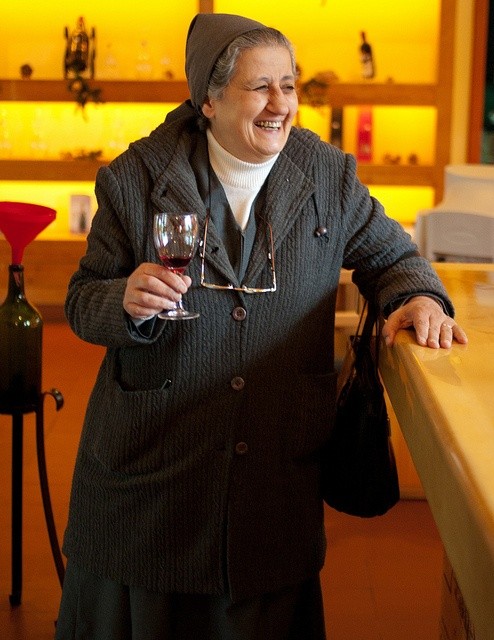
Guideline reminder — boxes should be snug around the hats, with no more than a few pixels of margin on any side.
[185,13,268,121]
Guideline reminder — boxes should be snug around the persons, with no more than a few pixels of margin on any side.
[56,13,468,640]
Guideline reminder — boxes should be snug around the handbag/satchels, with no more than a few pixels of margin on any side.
[320,250,423,520]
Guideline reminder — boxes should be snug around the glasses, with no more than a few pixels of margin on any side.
[200,208,277,295]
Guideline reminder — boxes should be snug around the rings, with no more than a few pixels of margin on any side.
[440,322,453,330]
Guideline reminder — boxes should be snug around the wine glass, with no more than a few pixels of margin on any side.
[152,213,200,321]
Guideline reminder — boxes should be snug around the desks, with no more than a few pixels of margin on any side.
[352,262,494,639]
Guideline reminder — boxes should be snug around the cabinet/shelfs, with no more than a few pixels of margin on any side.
[1,0,488,326]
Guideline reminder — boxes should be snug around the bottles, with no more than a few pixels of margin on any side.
[358,31,374,79]
[66,16,88,72]
[0,264,43,415]
